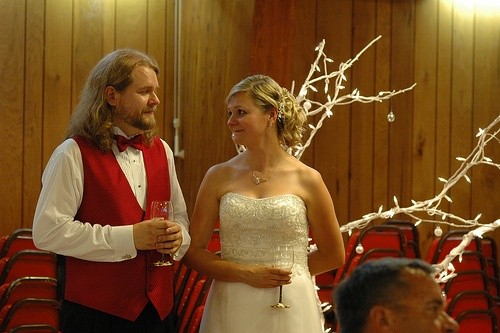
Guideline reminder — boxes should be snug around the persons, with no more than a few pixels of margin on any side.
[32,48,192,333]
[182,74,345,333]
[336,257,459,333]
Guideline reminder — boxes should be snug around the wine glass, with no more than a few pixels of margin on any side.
[270,243,294,309]
[152,200,175,266]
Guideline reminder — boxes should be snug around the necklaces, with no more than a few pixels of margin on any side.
[242,153,283,185]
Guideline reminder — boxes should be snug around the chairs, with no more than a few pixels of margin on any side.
[0,212,500,333]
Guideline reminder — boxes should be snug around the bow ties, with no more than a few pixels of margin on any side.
[111,134,144,153]
